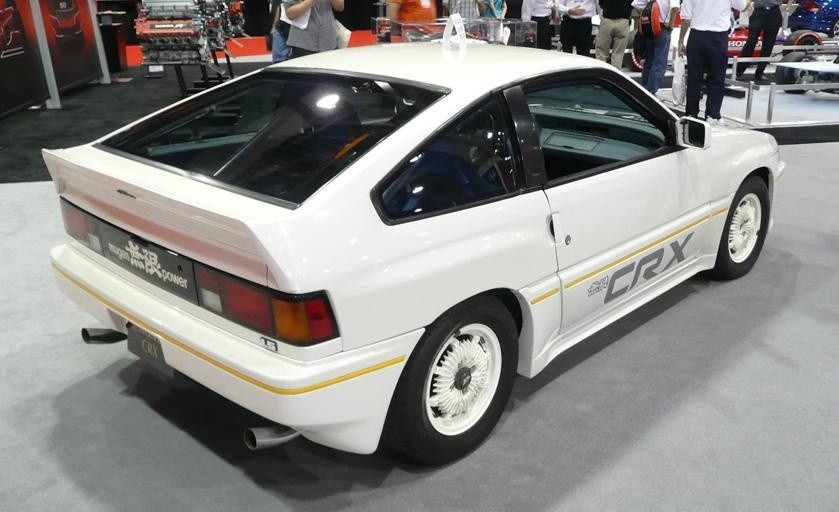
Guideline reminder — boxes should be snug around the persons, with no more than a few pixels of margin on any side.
[269,0,344,64]
[388,1,597,57]
[594,1,785,122]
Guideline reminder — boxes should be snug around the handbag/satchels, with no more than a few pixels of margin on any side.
[335,18,352,47]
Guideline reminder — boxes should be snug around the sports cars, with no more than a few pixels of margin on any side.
[589,2,824,72]
[774,48,839,96]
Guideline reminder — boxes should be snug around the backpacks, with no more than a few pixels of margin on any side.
[640,1,662,38]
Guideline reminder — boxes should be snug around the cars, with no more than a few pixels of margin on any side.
[787,0,839,38]
[35,12,788,465]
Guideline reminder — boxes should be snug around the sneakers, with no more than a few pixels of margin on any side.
[755,73,768,81]
